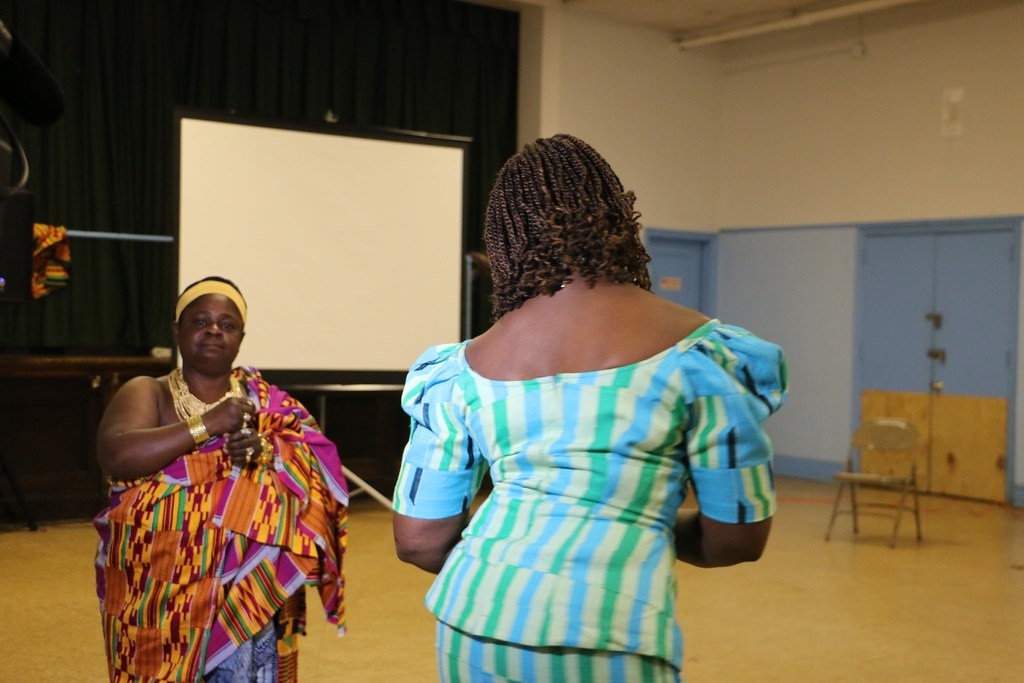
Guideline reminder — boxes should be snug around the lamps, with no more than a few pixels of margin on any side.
[676,1,902,78]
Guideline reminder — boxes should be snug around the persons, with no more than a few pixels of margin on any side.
[96,275,351,683]
[390,134,787,682]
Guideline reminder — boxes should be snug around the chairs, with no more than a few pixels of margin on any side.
[827,419,924,548]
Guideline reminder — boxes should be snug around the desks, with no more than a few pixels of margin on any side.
[0,351,167,421]
[277,385,407,440]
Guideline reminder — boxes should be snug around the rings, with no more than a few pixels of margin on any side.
[246,446,254,456]
[245,455,251,464]
[247,398,254,405]
[243,411,251,421]
[241,429,252,437]
[243,421,248,428]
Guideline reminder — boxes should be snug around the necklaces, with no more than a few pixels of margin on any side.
[169,366,245,423]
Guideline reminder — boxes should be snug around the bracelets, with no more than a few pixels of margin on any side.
[184,415,210,445]
